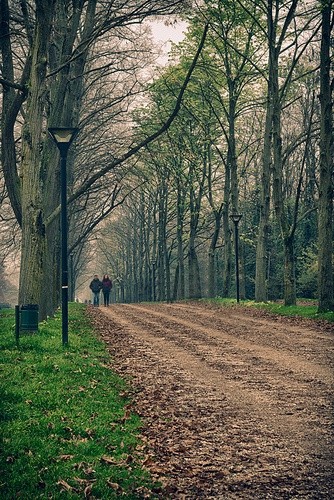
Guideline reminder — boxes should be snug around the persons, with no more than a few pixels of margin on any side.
[89,274,103,307]
[101,274,113,307]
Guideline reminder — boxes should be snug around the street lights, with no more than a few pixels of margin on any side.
[150,260,157,301]
[48,125,79,346]
[228,214,243,303]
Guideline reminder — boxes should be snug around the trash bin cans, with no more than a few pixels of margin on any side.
[19,302,39,336]
